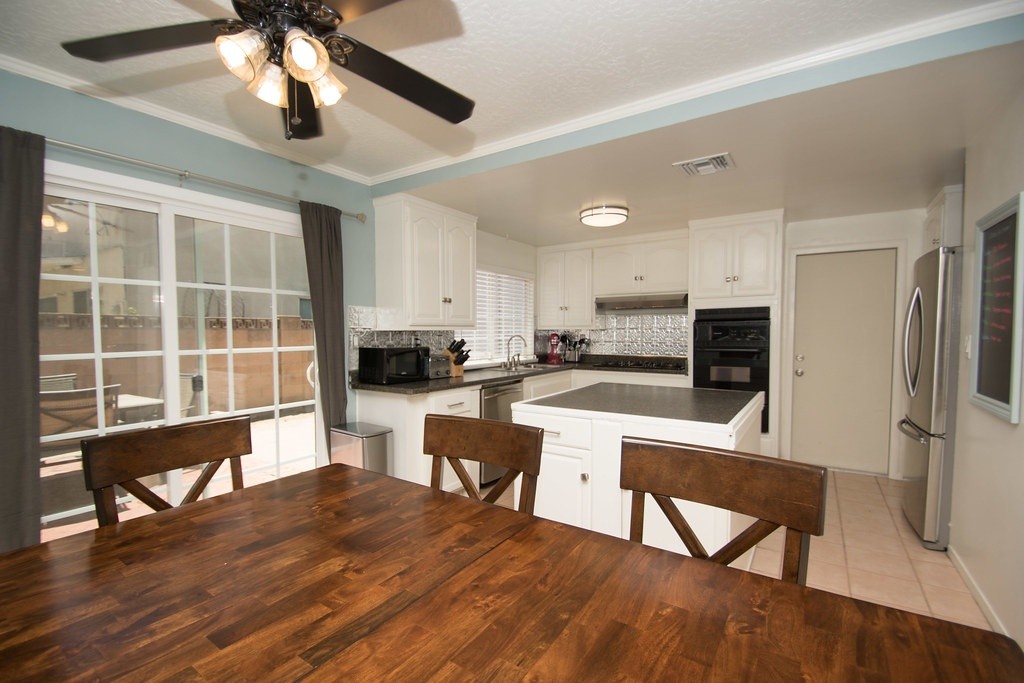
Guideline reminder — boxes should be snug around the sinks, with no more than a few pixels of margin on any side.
[505,363,561,371]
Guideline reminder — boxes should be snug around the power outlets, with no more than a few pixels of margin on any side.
[558,329,589,341]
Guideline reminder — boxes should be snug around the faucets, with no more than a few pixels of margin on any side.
[506,335,528,368]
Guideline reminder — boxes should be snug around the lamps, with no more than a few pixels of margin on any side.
[213,12,348,141]
[579,204,629,227]
[42,206,69,233]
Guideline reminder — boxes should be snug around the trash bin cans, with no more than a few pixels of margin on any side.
[330,422,395,477]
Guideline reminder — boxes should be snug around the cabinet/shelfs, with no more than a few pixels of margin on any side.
[355,385,482,498]
[372,192,477,331]
[593,236,690,298]
[522,369,687,401]
[688,215,786,299]
[538,248,606,330]
[511,411,761,571]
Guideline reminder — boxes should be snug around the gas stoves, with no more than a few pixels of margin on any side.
[593,359,684,370]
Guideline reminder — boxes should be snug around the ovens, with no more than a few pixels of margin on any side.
[692,307,771,434]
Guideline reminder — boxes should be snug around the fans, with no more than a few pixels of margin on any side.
[60,0,476,140]
[43,195,123,229]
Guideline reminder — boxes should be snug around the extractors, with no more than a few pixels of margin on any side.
[595,293,688,308]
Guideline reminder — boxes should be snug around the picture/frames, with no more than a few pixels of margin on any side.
[967,191,1024,424]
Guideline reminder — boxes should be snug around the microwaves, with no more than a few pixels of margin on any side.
[358,347,430,385]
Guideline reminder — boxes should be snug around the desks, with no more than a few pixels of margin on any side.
[115,394,163,426]
[0,463,1024,683]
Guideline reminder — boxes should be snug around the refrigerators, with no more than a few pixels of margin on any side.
[899,245,964,550]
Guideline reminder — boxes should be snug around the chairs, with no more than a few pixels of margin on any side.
[80,414,252,527]
[619,435,827,587]
[423,413,545,515]
[39,383,120,467]
[39,373,79,392]
[142,371,201,423]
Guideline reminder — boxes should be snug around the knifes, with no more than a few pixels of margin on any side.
[447,338,471,366]
